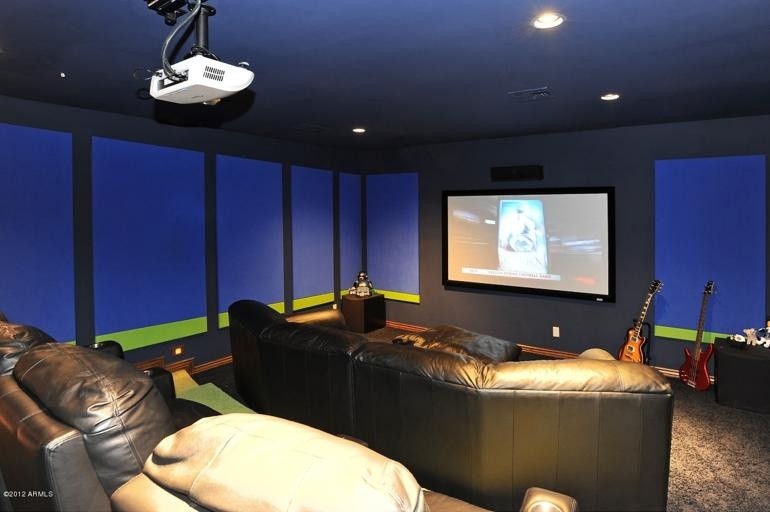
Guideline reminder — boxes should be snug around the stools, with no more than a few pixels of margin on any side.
[391,324,522,366]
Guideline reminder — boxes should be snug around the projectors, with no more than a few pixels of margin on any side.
[149,55,255,105]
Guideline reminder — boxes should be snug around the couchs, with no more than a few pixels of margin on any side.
[1,313,579,512]
[227,300,675,511]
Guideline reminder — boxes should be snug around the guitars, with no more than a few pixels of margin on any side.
[618,279,663,365]
[679,280,714,392]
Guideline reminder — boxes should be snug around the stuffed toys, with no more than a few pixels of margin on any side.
[743,328,757,346]
[755,320,770,348]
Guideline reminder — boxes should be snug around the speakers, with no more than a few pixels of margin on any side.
[491,165,544,181]
